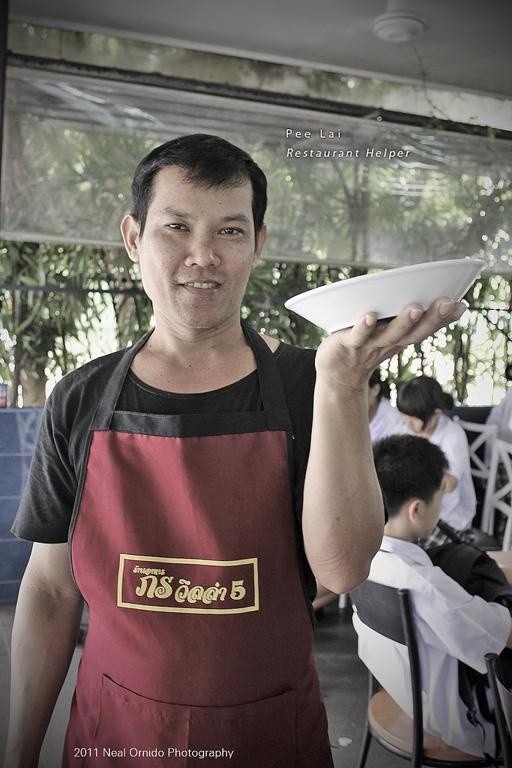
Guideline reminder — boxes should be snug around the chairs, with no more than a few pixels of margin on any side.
[453,413,512,554]
[348,582,511,767]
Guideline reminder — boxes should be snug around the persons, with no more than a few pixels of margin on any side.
[363,365,417,443]
[0,132,472,767]
[312,374,478,616]
[485,360,511,539]
[350,431,512,761]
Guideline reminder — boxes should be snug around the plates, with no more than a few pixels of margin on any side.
[283,257,485,331]
